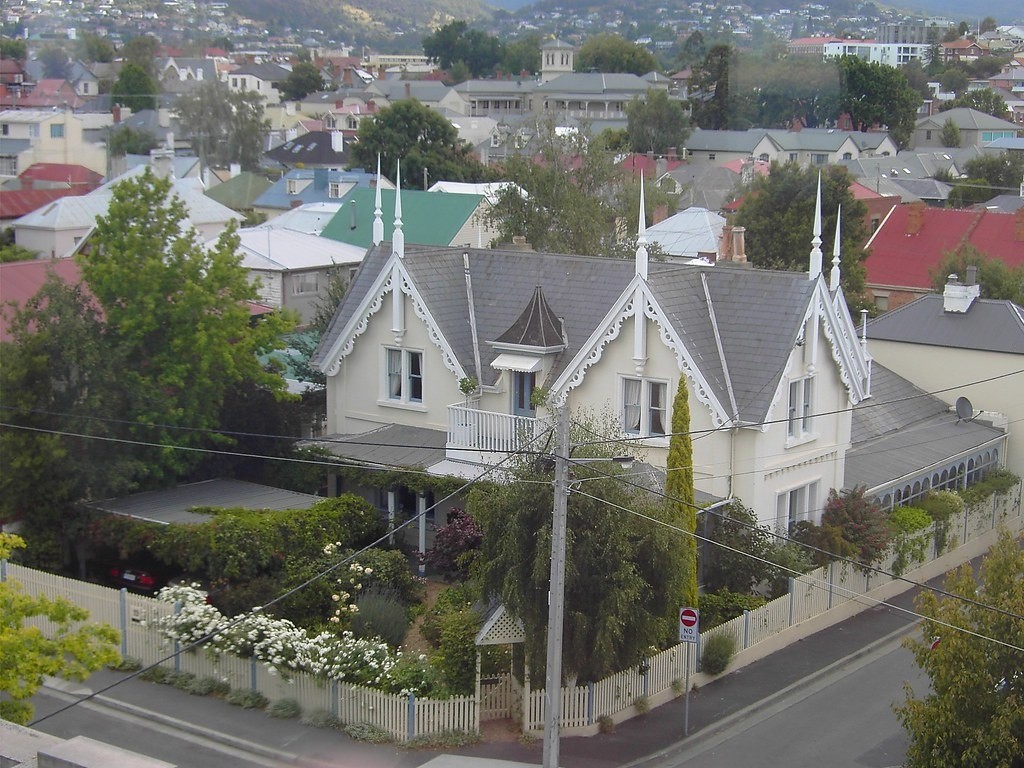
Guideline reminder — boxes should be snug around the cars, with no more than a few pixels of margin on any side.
[113,553,177,598]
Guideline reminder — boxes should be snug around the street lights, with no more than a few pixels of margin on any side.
[541,453,636,768]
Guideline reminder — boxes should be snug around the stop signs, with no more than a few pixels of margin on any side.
[681,609,697,627]
